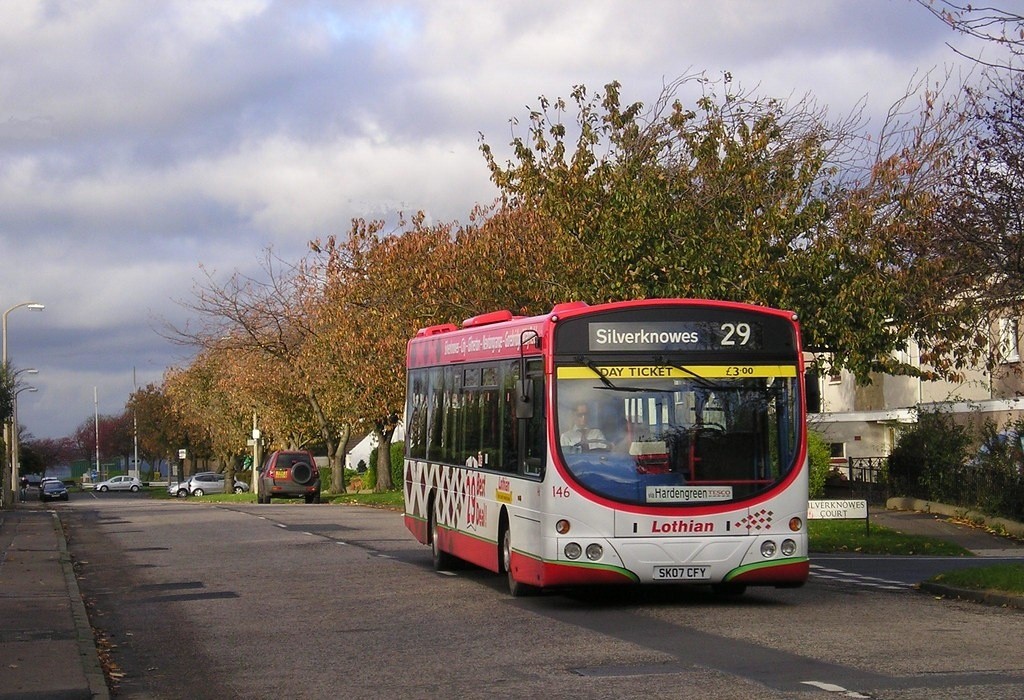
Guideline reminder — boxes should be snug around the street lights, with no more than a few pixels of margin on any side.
[12,368,39,492]
[15,386,38,437]
[2,302,45,506]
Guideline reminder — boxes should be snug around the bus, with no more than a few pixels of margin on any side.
[402,296,811,598]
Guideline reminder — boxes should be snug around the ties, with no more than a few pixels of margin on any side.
[577,429,589,454]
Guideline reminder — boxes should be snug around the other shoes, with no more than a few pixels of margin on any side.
[22,501,24,504]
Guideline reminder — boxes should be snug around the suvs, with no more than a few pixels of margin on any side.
[255,449,320,504]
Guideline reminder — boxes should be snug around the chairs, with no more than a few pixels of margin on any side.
[646,422,726,478]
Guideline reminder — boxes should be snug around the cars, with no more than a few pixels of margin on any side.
[40,481,69,502]
[40,476,57,488]
[166,478,190,497]
[963,431,1024,483]
[188,473,249,496]
[94,475,143,492]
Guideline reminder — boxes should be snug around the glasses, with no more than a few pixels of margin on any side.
[575,412,589,418]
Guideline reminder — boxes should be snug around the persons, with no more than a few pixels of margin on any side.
[560,401,608,453]
[20,476,30,504]
